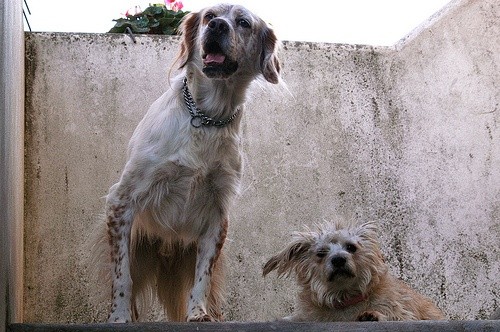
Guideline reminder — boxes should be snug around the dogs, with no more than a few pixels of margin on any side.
[263,219,450,322]
[88,3,281,326]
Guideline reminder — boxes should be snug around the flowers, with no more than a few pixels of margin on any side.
[107,0,190,36]
